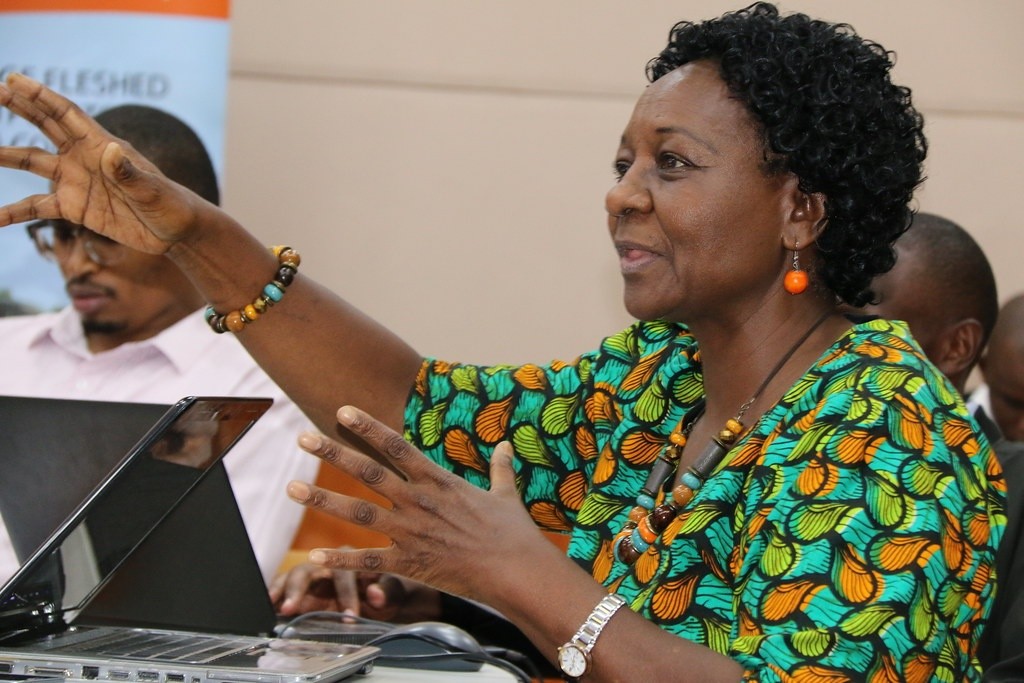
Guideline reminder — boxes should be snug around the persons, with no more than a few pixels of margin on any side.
[853,212,1023,682]
[0,2,1024,683]
[0,106,329,621]
[958,293,1024,447]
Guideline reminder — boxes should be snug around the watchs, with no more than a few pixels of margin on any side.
[556,593,628,683]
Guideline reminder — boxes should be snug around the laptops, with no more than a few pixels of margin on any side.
[0,396,380,682]
[0,396,394,645]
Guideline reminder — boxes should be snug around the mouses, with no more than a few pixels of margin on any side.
[369,620,486,671]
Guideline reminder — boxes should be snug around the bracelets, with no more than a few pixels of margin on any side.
[203,243,299,337]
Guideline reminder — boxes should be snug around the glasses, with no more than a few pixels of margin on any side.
[27,218,125,267]
